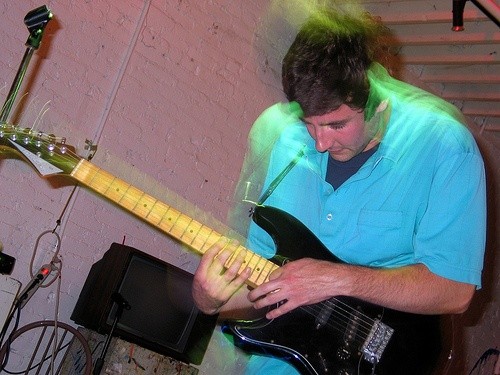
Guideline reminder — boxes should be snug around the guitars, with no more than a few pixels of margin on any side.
[0,121,443,375]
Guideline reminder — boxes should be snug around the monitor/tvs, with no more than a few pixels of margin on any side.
[70,242,199,354]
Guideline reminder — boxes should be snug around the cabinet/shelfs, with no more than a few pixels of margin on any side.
[59,328,199,375]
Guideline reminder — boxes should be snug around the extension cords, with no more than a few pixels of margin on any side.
[14,264,52,309]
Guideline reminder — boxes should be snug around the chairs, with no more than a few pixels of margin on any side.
[0,320,92,375]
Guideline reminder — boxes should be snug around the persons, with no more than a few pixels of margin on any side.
[192,2,488,375]
